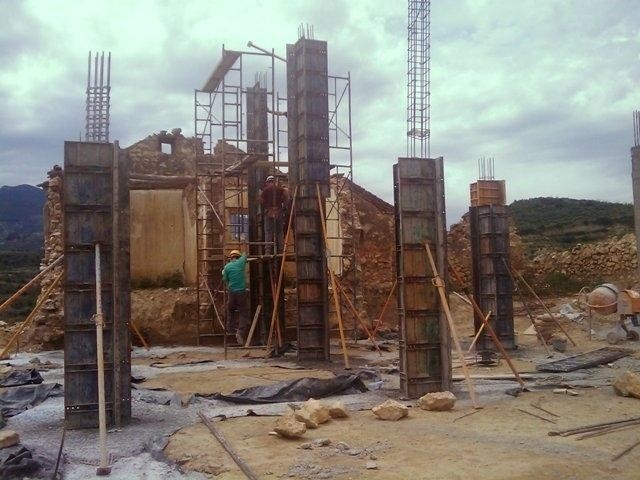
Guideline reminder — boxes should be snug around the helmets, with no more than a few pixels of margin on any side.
[230,250,241,256]
[266,176,274,180]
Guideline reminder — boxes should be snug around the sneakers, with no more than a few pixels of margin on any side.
[235,333,244,344]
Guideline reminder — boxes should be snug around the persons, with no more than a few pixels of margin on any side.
[222,232,251,345]
[256,175,288,256]
